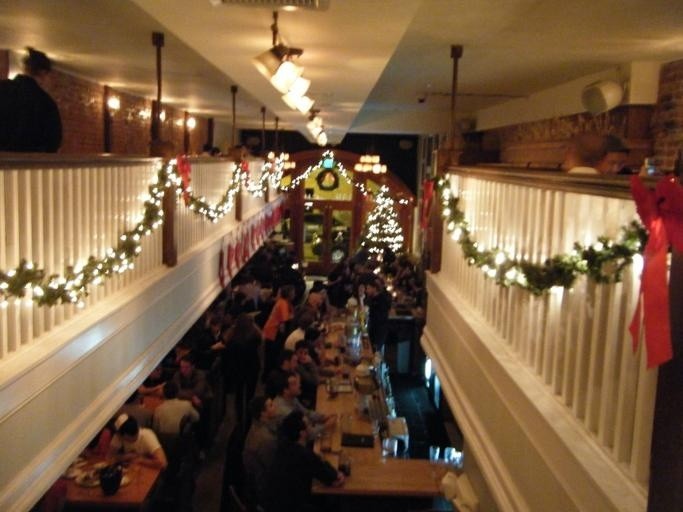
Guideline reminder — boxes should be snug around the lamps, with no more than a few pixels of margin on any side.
[106,96,119,117]
[252,11,315,116]
[267,151,296,170]
[306,109,328,147]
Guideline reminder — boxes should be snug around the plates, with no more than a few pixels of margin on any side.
[62,456,130,488]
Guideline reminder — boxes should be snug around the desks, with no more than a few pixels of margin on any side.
[66,465,161,512]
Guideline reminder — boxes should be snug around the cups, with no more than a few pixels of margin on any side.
[333,356,341,367]
[337,453,351,476]
[341,368,349,384]
[325,378,338,397]
[321,430,332,452]
[444,446,464,470]
[428,445,441,464]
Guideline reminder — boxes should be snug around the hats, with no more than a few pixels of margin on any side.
[114,414,128,429]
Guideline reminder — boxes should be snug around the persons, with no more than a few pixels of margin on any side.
[0,50,63,153]
[81,242,428,512]
[568,130,633,177]
[201,144,251,159]
